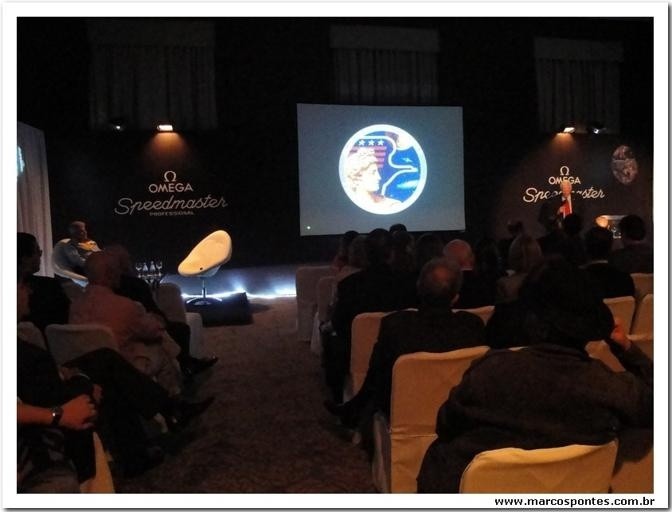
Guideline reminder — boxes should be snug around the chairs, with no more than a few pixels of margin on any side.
[51,230,233,309]
[17,277,187,494]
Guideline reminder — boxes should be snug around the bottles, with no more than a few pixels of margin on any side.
[142,262,149,277]
[150,261,156,276]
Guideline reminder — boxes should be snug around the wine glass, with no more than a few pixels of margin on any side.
[135,262,143,279]
[156,260,164,277]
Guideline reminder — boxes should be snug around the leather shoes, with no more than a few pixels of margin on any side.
[180,395,215,429]
[124,459,159,479]
[192,356,219,374]
[324,399,354,427]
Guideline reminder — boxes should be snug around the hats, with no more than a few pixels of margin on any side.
[520,276,615,340]
[444,241,476,272]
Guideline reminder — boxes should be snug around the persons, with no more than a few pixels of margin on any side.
[17,233,220,493]
[328,211,651,493]
[538,180,588,229]
[65,220,100,271]
[345,148,402,209]
